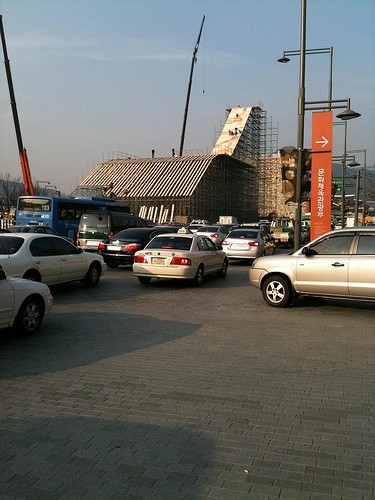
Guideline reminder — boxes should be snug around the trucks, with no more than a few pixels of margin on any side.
[269,218,296,248]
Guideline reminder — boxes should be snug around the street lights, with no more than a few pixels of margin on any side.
[277,46,334,112]
[340,176,357,229]
[293,98,361,250]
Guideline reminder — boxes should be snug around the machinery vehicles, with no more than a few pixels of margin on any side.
[0,15,37,196]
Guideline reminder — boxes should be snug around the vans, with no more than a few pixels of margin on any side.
[76,210,149,253]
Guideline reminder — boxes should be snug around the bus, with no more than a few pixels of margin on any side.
[302,213,334,227]
[17,195,132,241]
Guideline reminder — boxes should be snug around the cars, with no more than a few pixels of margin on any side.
[249,227,375,306]
[222,228,275,260]
[96,227,193,269]
[189,215,274,246]
[0,264,55,336]
[334,223,342,230]
[133,227,229,287]
[8,225,74,245]
[0,232,109,289]
